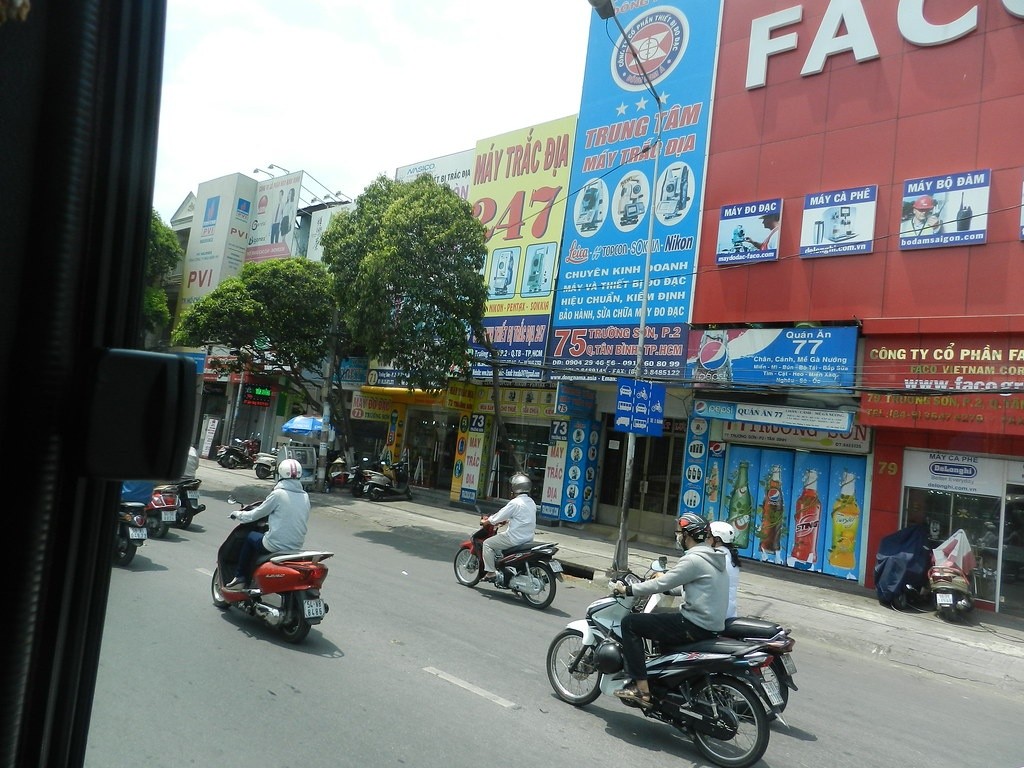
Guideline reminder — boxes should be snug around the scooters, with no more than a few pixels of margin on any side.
[113,473,206,567]
[883,529,979,620]
[349,458,412,500]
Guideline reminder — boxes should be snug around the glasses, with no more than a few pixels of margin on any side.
[674,529,685,536]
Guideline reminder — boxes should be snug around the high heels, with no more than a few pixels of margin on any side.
[225,578,246,591]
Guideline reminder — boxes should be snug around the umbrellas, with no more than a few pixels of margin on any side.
[282,415,336,447]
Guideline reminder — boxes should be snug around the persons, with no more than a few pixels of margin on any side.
[975,522,1019,564]
[160,446,199,486]
[120,480,154,508]
[271,188,296,243]
[226,459,312,590]
[900,195,943,238]
[744,213,781,250]
[478,475,537,582]
[614,513,742,709]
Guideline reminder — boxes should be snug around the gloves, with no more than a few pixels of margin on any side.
[230,511,243,520]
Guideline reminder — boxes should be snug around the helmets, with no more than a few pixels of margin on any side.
[511,473,531,493]
[278,459,303,479]
[679,512,710,540]
[710,521,736,543]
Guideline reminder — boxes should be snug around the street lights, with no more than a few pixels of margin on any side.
[591,0,664,573]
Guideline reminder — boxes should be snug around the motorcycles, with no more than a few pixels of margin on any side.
[216,433,262,470]
[621,556,797,722]
[210,459,333,642]
[251,452,277,478]
[546,582,771,768]
[452,475,564,609]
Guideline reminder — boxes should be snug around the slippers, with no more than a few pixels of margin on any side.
[479,576,497,583]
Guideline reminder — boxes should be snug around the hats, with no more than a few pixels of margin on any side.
[759,214,780,219]
[913,196,933,209]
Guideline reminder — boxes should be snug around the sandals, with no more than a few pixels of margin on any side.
[614,685,654,710]
